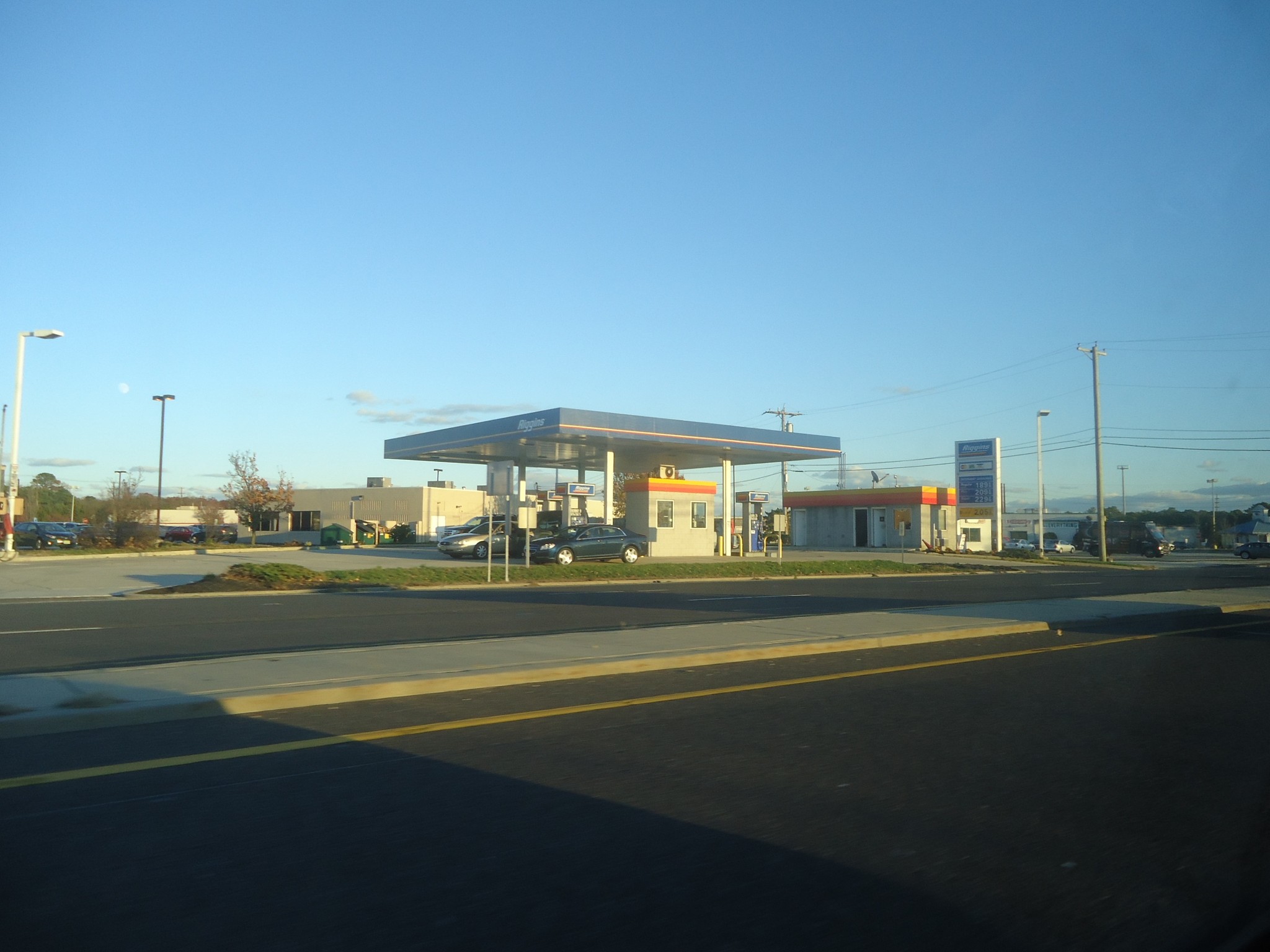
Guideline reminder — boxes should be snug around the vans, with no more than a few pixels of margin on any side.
[1088,520,1171,559]
[1172,541,1188,550]
[714,516,744,548]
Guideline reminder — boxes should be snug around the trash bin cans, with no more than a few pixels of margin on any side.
[391,522,417,544]
[355,519,376,544]
[378,524,394,544]
[321,523,353,546]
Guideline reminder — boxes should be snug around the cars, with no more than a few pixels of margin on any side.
[1043,539,1076,554]
[1032,539,1057,553]
[1233,541,1270,559]
[436,521,552,560]
[165,526,203,543]
[522,523,649,566]
[12,522,78,550]
[1004,539,1035,554]
[0,522,21,549]
[190,524,206,529]
[56,521,175,546]
[992,536,1011,549]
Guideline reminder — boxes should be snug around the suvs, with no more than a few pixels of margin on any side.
[190,524,238,545]
[435,514,517,539]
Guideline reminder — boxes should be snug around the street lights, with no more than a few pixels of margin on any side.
[434,469,443,481]
[115,470,127,498]
[152,395,175,538]
[1206,478,1217,531]
[1036,409,1051,558]
[1117,465,1129,515]
[2,329,65,560]
